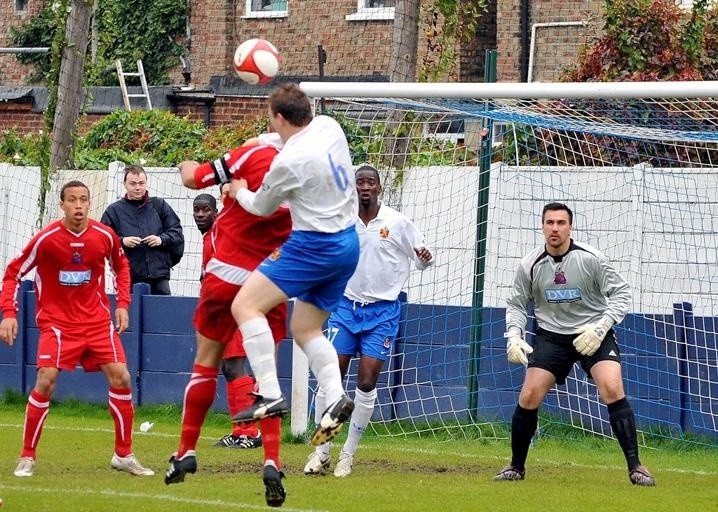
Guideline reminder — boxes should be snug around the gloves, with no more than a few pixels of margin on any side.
[504,327,533,366]
[572,317,614,356]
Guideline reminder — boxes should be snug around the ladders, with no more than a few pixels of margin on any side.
[115,59,152,113]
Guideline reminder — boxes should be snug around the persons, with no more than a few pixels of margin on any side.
[493,204,655,485]
[221,182,230,204]
[229,84,359,446]
[165,119,286,507]
[304,165,433,477]
[1,181,155,477]
[194,195,264,447]
[101,166,183,295]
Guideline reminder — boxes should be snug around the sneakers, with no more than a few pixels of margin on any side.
[231,392,288,424]
[14,457,35,477]
[110,452,154,475]
[210,430,262,449]
[304,395,354,477]
[494,466,525,480]
[264,459,285,507]
[629,465,655,486]
[165,449,196,485]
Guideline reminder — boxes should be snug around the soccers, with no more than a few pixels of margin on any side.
[234,38,281,85]
